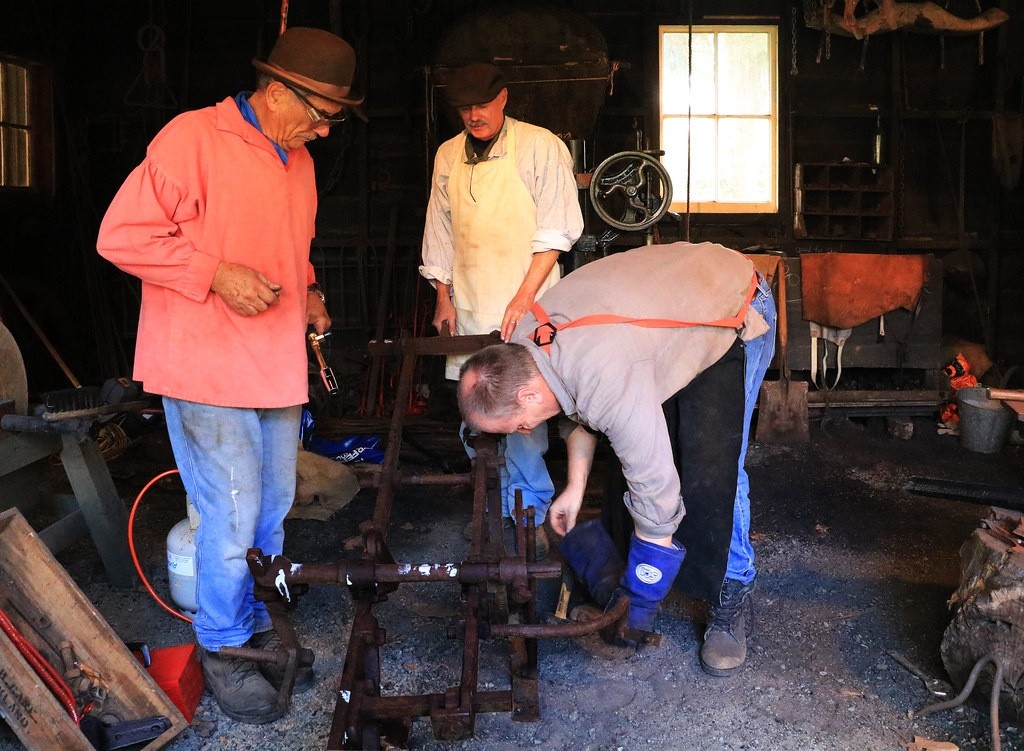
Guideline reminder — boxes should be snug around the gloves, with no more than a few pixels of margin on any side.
[598,531,686,646]
[558,517,627,610]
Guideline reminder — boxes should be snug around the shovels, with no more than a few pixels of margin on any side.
[755,259,814,443]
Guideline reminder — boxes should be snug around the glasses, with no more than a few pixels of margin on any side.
[273,77,348,126]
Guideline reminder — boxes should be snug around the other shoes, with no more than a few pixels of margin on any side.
[464,512,513,541]
[515,524,550,560]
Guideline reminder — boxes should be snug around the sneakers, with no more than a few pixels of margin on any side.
[199,648,284,724]
[700,569,758,678]
[250,629,316,694]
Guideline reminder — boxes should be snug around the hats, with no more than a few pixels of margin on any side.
[446,63,508,107]
[252,26,364,105]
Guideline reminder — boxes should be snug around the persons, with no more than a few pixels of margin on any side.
[96,25,365,725]
[419,61,585,563]
[456,240,778,678]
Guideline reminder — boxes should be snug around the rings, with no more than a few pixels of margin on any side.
[516,323,518,325]
[510,320,515,324]
